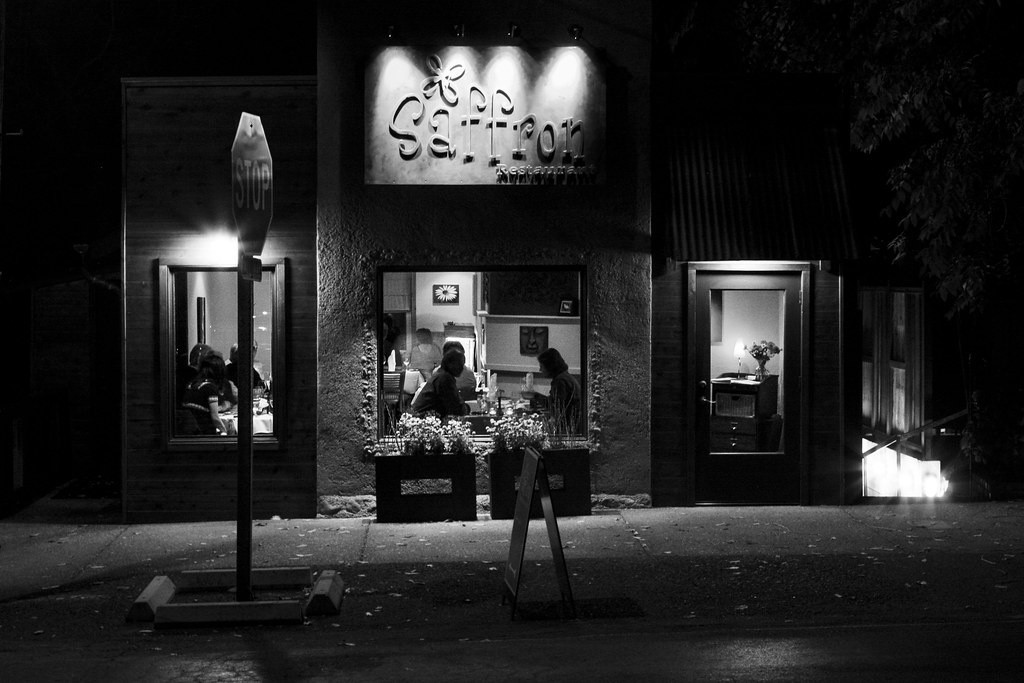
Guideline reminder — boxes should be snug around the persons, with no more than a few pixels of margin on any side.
[177,340,263,438]
[519,348,585,436]
[436,341,476,399]
[409,328,443,372]
[411,350,471,418]
[519,326,548,352]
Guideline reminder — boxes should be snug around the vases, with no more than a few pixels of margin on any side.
[489,450,593,517]
[752,355,770,380]
[374,454,476,522]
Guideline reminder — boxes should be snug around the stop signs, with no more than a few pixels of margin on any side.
[227,112,276,259]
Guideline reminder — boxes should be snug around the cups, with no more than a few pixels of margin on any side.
[253,389,261,402]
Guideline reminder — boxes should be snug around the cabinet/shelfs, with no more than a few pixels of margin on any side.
[711,373,779,453]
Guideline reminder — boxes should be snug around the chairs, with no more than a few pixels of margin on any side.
[176,409,203,436]
[383,372,409,434]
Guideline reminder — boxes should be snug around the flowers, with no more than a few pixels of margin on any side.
[744,340,783,377]
[377,403,477,455]
[486,384,583,453]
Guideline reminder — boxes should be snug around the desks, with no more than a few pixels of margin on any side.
[220,409,273,435]
[446,398,523,435]
